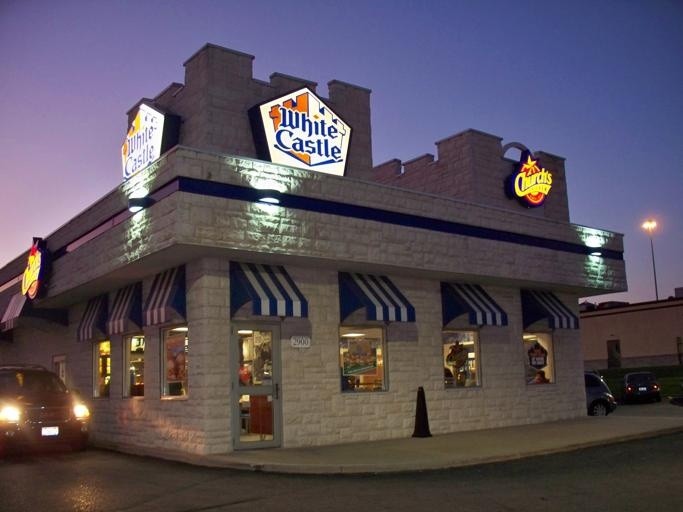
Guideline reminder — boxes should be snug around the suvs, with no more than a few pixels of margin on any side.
[0,361,90,456]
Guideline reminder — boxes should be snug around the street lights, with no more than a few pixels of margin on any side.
[641,219,659,301]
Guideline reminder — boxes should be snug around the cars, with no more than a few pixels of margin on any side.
[584,373,618,417]
[622,371,663,405]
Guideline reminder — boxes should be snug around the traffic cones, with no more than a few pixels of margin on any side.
[412,387,434,439]
